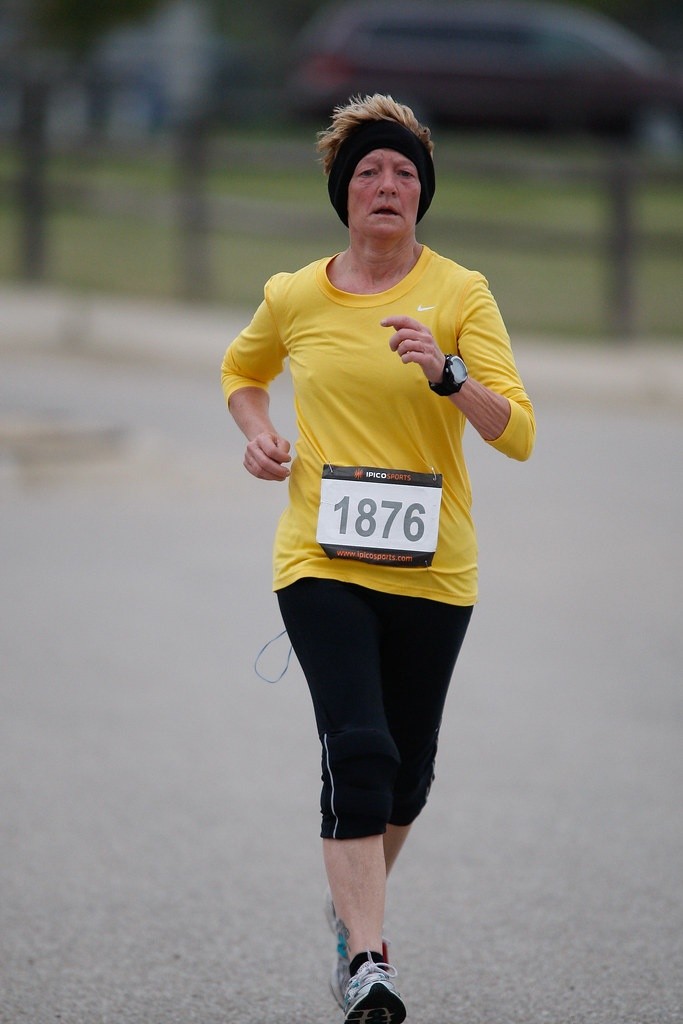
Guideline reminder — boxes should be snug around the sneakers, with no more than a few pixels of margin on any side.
[342,952,407,1024]
[324,894,354,1013]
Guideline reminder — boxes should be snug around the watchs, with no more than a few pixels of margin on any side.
[427,353,467,397]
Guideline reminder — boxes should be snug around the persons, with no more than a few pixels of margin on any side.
[221,92,534,1024]
